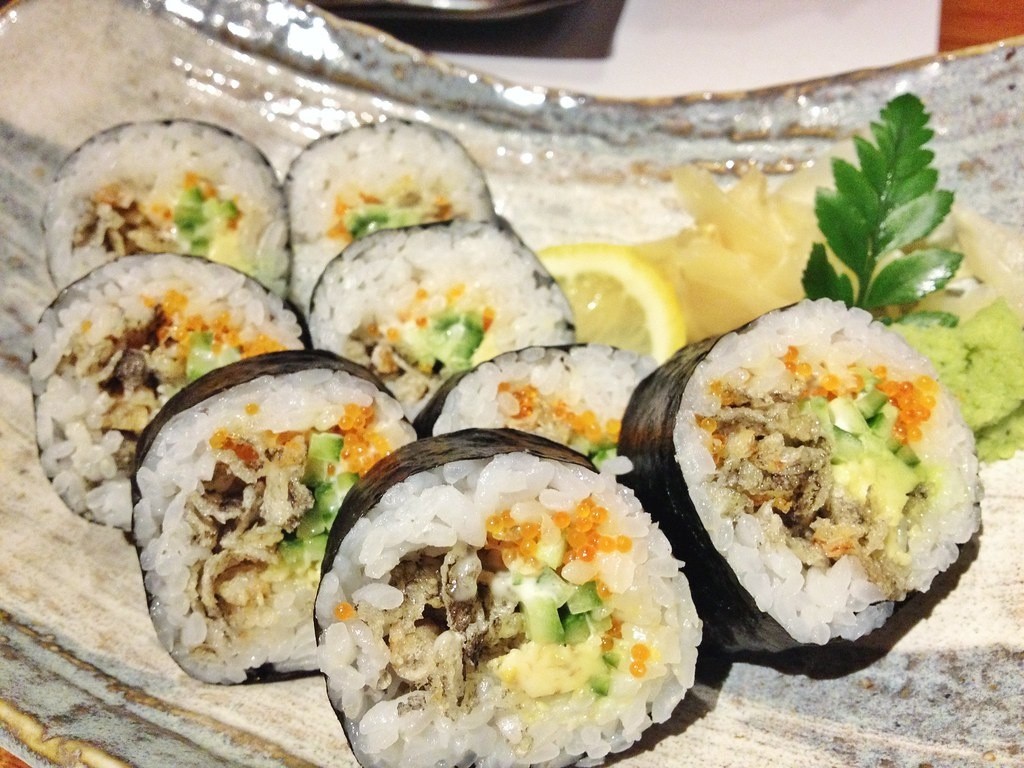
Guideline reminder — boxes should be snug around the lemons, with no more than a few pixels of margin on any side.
[533,244,687,363]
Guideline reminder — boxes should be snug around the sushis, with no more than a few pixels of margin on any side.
[29,116,986,768]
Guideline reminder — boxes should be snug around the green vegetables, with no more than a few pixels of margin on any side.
[798,95,964,330]
